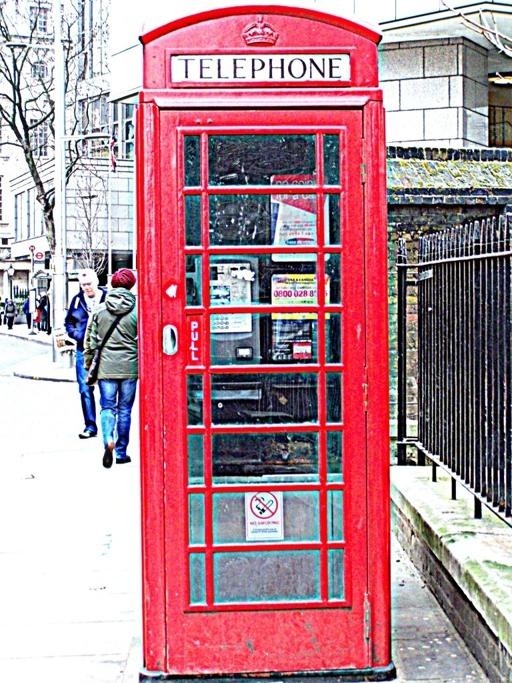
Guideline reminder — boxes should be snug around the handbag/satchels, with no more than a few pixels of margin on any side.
[86,349,100,386]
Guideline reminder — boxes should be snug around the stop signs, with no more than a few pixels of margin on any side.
[34,248,46,260]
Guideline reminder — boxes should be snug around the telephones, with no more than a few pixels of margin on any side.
[185,254,262,365]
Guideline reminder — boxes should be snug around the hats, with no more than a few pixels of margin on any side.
[111,269,135,288]
[80,271,95,283]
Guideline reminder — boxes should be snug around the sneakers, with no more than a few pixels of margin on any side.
[103,447,112,468]
[116,456,131,463]
[79,431,96,438]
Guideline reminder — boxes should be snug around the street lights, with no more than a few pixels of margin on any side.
[5,41,110,329]
[7,263,16,300]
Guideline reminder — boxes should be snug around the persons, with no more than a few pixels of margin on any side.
[0,291,50,336]
[81,265,138,466]
[63,266,104,437]
